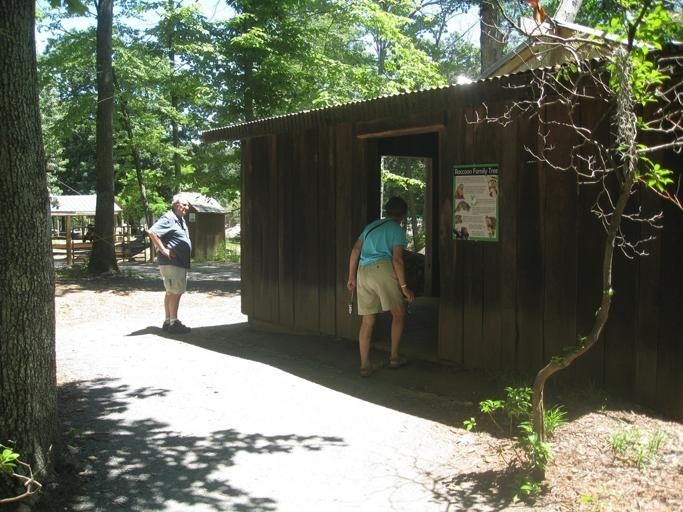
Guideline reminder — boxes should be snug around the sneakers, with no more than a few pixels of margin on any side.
[163,320,191,333]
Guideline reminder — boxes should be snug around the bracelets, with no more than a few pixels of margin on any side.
[399,280,409,287]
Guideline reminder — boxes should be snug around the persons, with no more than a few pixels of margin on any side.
[347,196,416,378]
[148,195,191,334]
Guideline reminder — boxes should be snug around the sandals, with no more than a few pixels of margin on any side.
[389,356,407,368]
[360,361,385,377]
